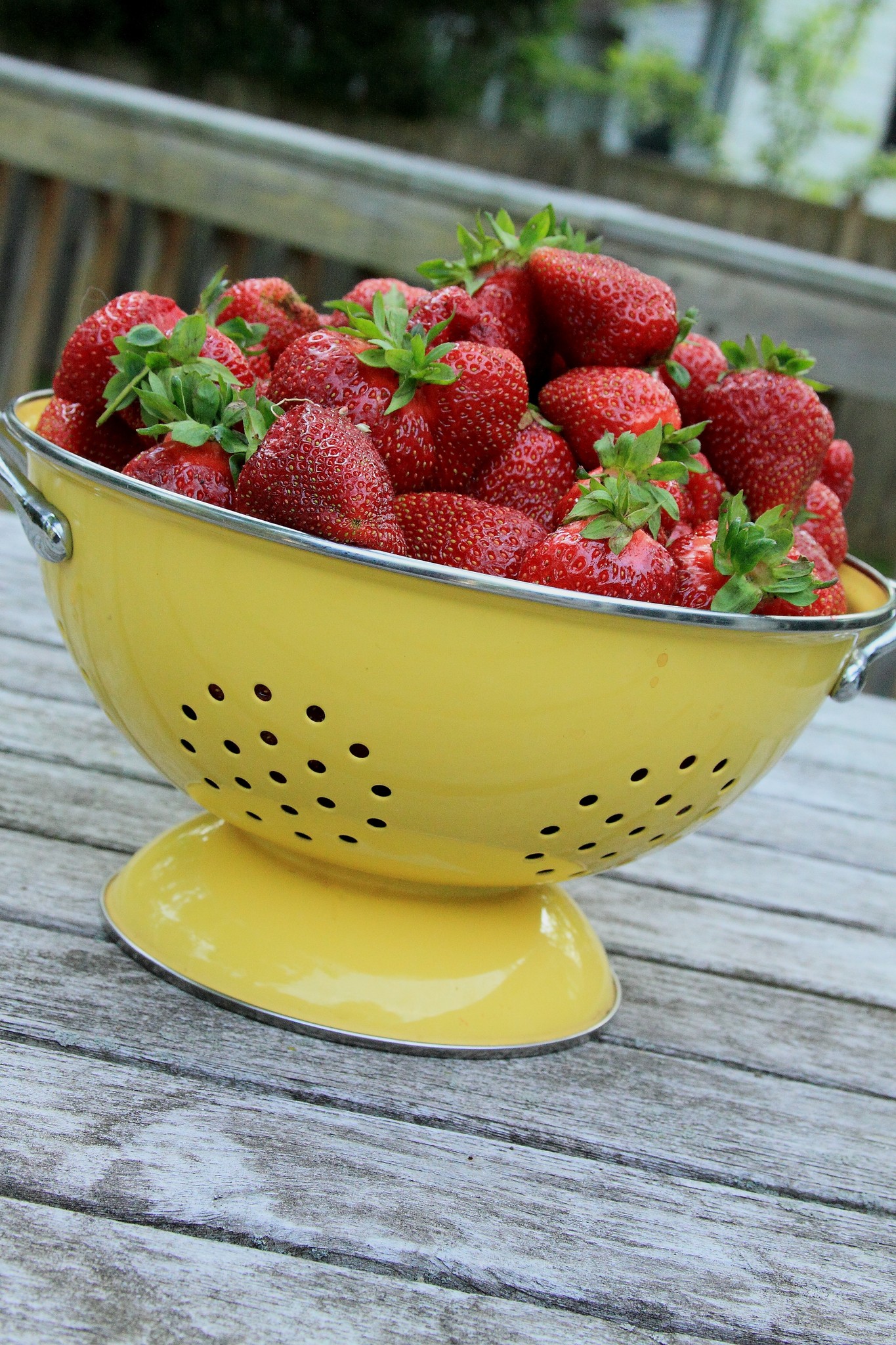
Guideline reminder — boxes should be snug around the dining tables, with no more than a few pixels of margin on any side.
[0,512,893,1343]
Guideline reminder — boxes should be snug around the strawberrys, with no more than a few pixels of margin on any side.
[31,197,853,619]
